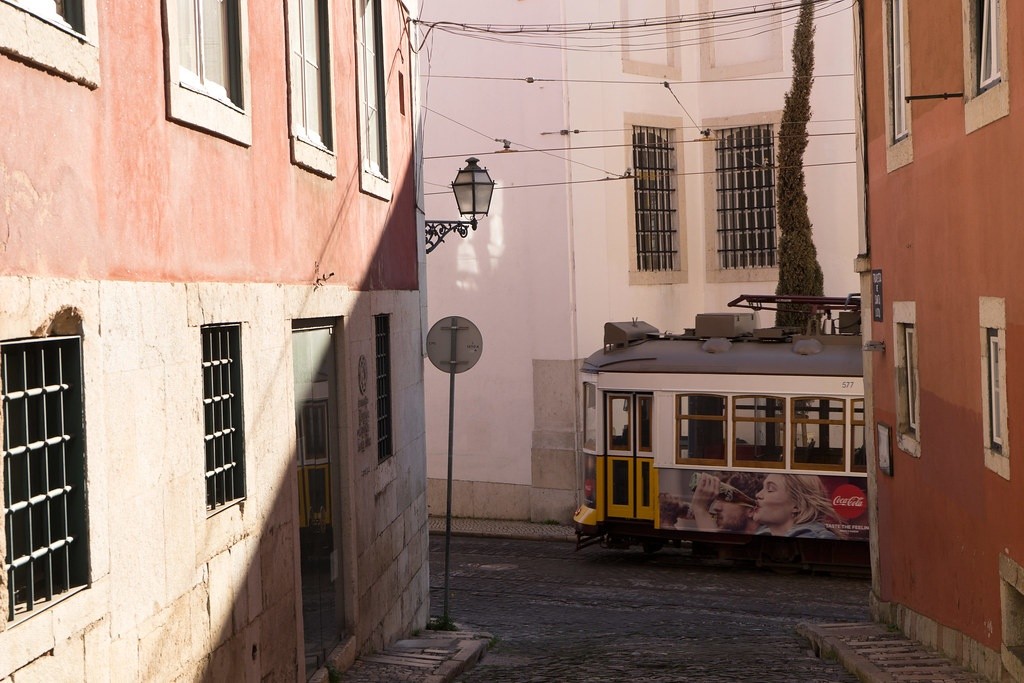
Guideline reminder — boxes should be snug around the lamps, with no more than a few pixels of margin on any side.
[425,157,495,255]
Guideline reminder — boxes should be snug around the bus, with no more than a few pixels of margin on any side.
[572,311,868,568]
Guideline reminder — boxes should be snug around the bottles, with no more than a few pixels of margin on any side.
[659,500,719,520]
[689,472,758,509]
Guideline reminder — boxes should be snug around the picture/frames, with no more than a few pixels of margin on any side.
[877,421,894,477]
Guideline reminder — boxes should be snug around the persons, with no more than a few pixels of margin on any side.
[659,473,840,538]
